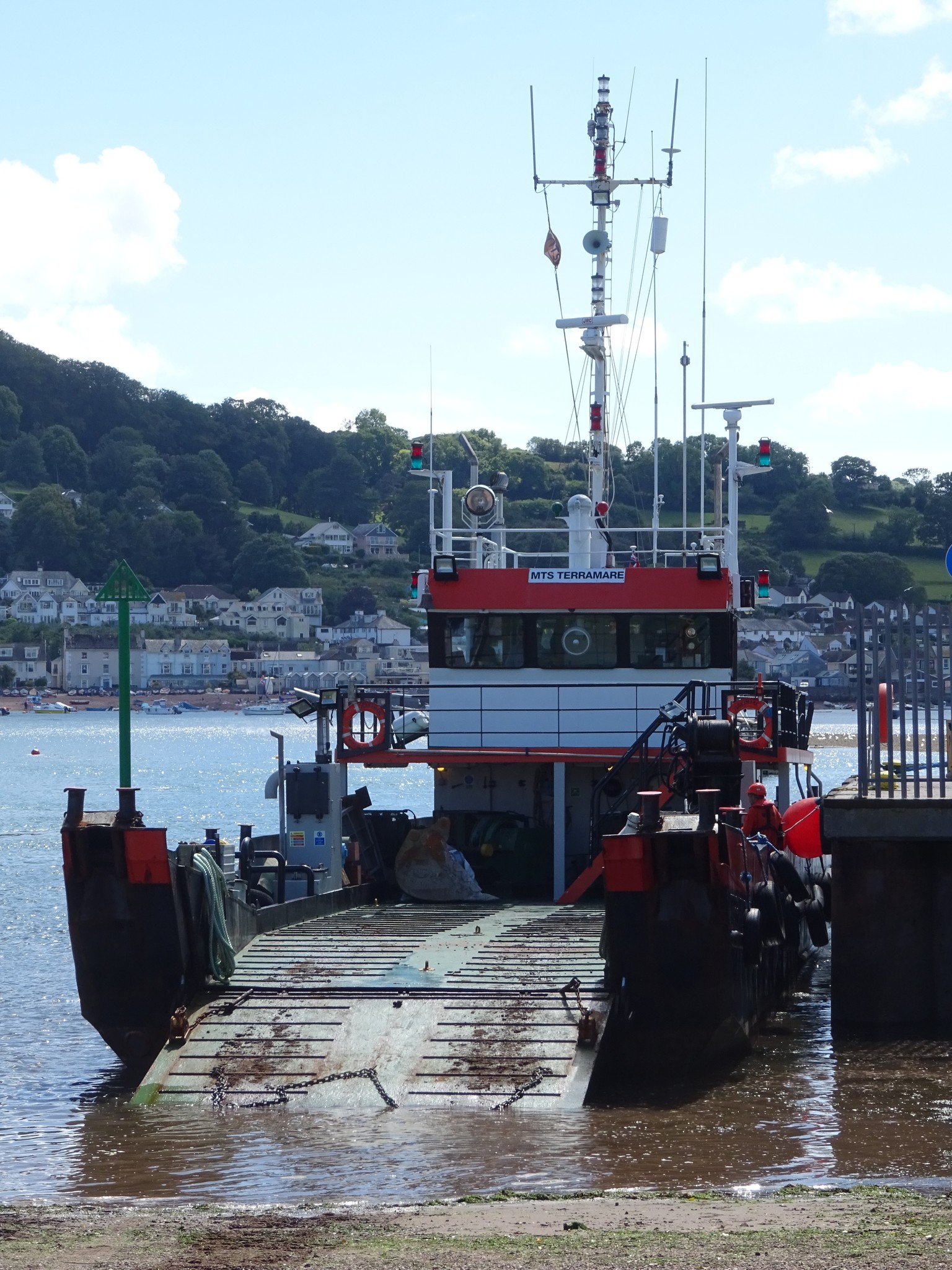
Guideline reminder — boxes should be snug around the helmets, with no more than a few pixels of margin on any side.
[746,783,767,797]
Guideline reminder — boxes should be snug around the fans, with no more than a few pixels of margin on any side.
[562,626,593,656]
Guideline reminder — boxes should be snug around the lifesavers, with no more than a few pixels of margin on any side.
[342,701,386,750]
[727,697,773,749]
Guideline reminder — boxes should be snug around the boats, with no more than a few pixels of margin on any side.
[287,52,824,814]
[864,701,951,720]
[2,686,231,697]
[171,700,208,712]
[805,699,810,706]
[68,699,91,705]
[85,705,113,711]
[142,699,177,714]
[823,700,853,710]
[32,702,70,714]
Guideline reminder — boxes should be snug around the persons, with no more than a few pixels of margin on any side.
[742,782,783,852]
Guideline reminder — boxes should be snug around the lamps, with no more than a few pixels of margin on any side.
[433,554,458,581]
[287,698,315,718]
[460,485,500,529]
[319,688,339,709]
[697,553,722,580]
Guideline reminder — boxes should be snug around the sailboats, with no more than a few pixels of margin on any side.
[241,643,288,716]
